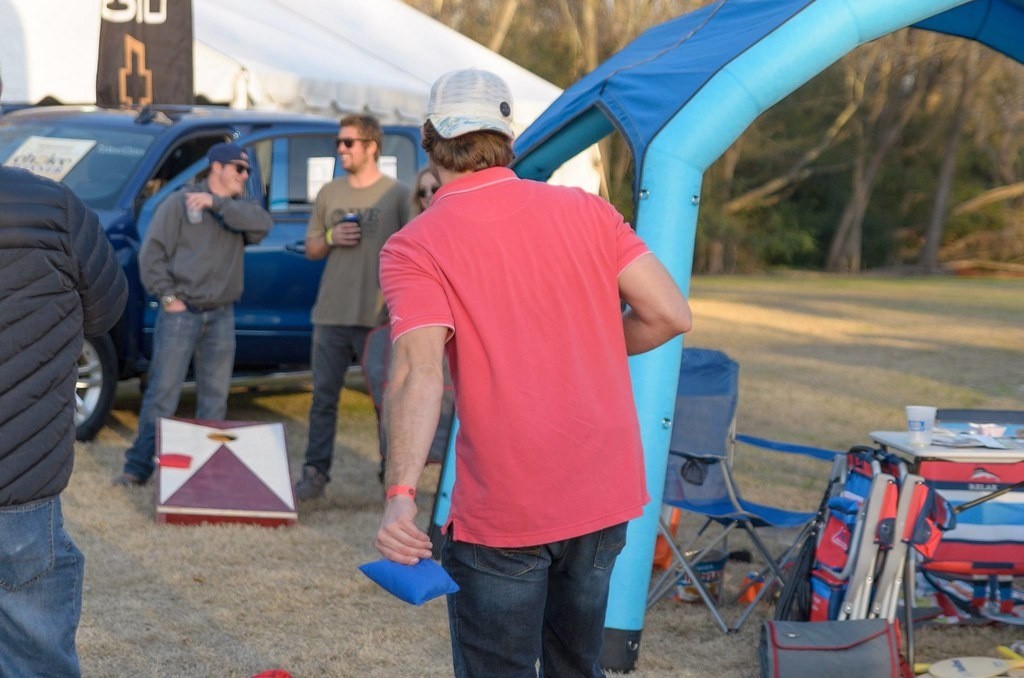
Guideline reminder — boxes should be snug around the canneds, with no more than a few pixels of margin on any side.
[342,212,360,227]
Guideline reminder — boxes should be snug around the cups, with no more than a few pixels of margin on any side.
[186,198,203,225]
[908,403,940,445]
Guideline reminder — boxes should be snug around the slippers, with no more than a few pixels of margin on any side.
[419,185,439,198]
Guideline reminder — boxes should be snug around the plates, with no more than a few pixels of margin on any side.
[968,421,1006,439]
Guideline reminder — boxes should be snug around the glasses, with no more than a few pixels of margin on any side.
[336,137,372,148]
[219,160,251,174]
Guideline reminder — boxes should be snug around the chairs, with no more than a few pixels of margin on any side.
[646,347,845,635]
[804,444,949,674]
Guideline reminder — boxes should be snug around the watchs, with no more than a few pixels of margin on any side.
[159,295,177,305]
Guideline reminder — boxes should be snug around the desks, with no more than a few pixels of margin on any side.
[868,429,1024,609]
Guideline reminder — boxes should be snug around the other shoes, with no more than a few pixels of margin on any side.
[292,465,331,500]
[112,474,144,487]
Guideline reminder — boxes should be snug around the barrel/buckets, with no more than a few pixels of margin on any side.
[675,548,729,602]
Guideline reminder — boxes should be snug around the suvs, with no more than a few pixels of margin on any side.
[0,101,431,441]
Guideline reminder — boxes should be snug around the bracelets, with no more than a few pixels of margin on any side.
[324,227,337,247]
[383,483,419,505]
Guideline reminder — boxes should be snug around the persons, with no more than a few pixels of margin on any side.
[375,68,693,677]
[291,114,416,502]
[0,70,129,677]
[122,144,272,484]
[406,163,443,223]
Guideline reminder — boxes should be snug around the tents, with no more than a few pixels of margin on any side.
[425,2,1024,673]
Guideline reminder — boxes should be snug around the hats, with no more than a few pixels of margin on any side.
[209,143,251,162]
[424,67,516,138]
